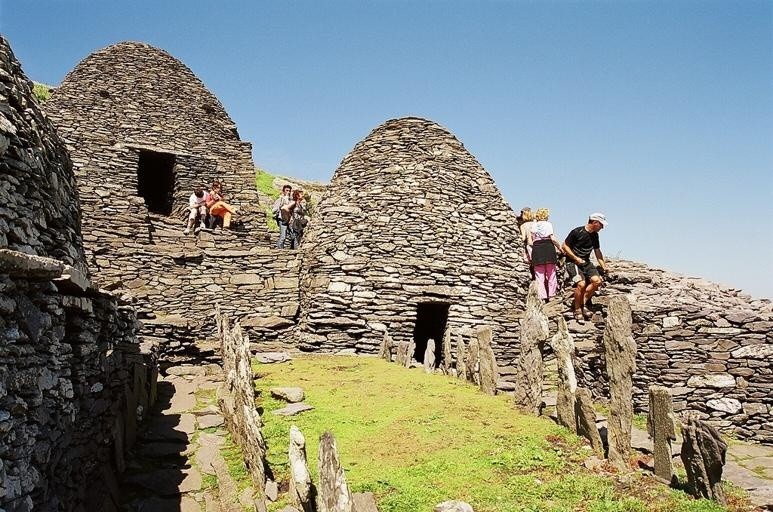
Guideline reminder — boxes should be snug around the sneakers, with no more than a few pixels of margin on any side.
[200,223,205,228]
[183,228,189,234]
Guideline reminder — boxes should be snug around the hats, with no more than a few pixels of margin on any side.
[589,213,608,226]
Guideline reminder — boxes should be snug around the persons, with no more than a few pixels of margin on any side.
[182,189,210,235]
[283,190,305,251]
[205,182,247,232]
[561,212,607,321]
[530,208,566,304]
[272,185,292,249]
[517,207,536,262]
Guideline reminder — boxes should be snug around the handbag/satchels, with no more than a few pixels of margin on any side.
[281,210,290,226]
[292,218,308,230]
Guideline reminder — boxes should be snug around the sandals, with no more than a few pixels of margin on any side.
[574,304,593,320]
